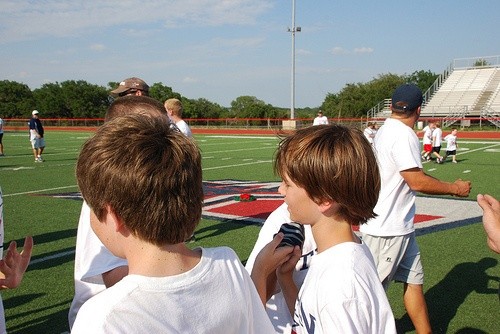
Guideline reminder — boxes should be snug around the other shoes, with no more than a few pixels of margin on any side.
[35,159,38,162]
[0,153,4,156]
[37,157,43,162]
[452,160,457,163]
[421,152,444,163]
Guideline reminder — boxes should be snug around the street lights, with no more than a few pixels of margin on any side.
[288,0,301,120]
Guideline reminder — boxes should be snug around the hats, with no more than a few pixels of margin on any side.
[318,110,322,113]
[32,110,39,115]
[392,83,423,110]
[111,78,149,94]
[368,122,375,126]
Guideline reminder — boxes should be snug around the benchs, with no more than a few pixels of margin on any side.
[422,67,500,114]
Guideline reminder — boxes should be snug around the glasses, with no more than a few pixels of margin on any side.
[119,90,138,96]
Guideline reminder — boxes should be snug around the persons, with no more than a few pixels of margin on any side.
[0,119,4,156]
[111,77,150,96]
[0,188,34,334]
[27,109,46,164]
[355,84,472,334]
[266,124,396,334]
[69,95,172,334]
[476,192,500,255]
[421,122,459,164]
[312,109,327,124]
[70,113,294,334]
[365,119,379,143]
[244,201,316,333]
[165,98,192,137]
